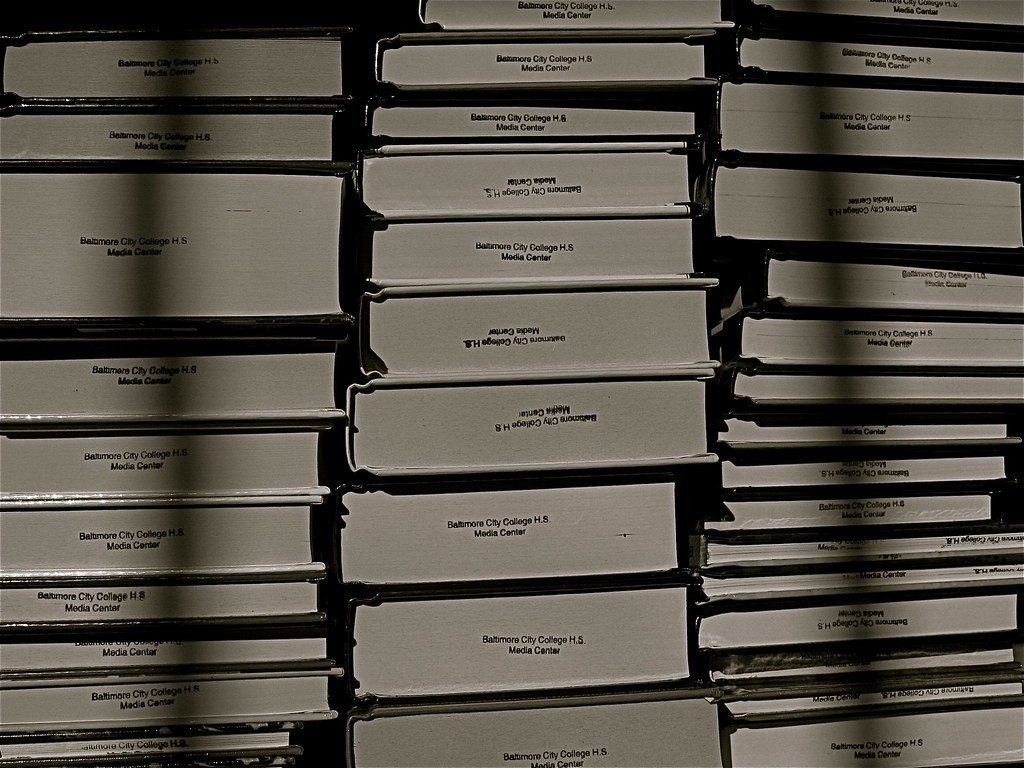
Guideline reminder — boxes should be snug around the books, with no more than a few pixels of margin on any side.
[0,0,1024,768]
